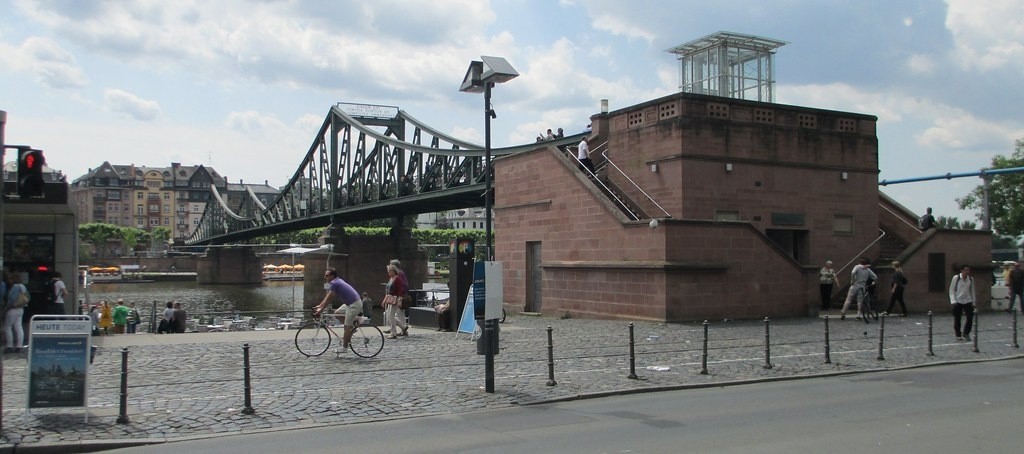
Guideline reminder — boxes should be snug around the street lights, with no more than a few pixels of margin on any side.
[458,52,519,395]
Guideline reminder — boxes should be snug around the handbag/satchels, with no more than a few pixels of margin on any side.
[902,277,908,285]
[12,287,29,308]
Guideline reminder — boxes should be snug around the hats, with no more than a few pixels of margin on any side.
[1003,262,1009,266]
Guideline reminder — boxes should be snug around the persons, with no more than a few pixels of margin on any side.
[948,265,976,339]
[46,272,68,321]
[534,126,599,178]
[300,165,493,213]
[362,259,449,339]
[1,266,29,354]
[311,267,363,351]
[921,208,936,231]
[839,256,908,320]
[78,299,187,334]
[992,261,1024,314]
[819,260,840,311]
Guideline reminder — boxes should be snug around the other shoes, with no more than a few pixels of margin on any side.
[333,346,348,353]
[383,295,397,304]
[840,311,845,320]
[386,334,397,339]
[955,336,963,340]
[963,334,971,341]
[856,317,863,321]
[435,327,449,332]
[398,331,408,336]
[383,330,391,333]
[401,294,412,309]
[881,311,889,317]
[15,348,24,352]
[4,347,14,353]
[899,313,908,317]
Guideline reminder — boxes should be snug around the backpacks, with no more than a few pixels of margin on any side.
[918,214,929,229]
[44,280,62,303]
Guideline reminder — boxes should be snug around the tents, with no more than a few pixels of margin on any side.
[87,266,119,275]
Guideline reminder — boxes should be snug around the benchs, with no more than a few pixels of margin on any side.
[407,306,440,328]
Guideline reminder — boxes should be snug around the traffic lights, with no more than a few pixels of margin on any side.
[16,147,47,199]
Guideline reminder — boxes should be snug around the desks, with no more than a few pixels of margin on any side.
[409,288,450,308]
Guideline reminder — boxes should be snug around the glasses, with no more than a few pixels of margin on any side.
[325,275,329,277]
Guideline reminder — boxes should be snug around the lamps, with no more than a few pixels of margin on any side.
[649,219,658,230]
[651,164,657,172]
[842,172,848,180]
[725,164,732,171]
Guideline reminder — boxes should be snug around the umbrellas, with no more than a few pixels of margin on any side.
[264,262,305,273]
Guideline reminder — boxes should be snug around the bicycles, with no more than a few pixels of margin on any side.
[862,280,880,323]
[294,307,394,358]
